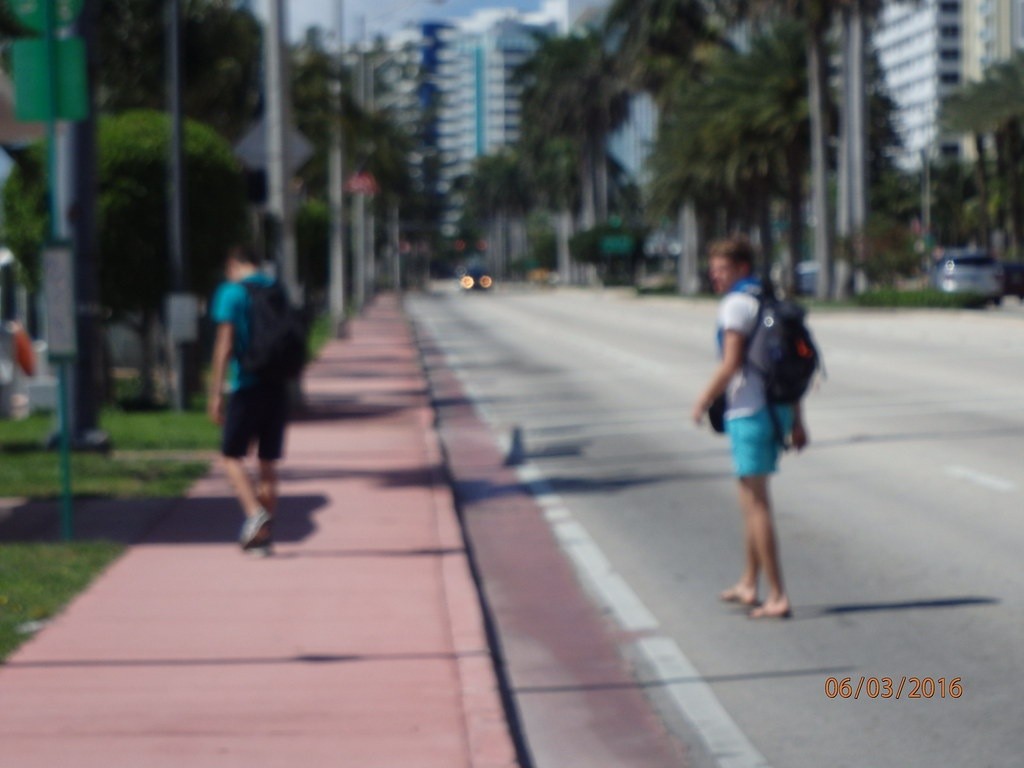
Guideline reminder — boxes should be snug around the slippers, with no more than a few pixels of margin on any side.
[719,590,760,607]
[750,606,789,618]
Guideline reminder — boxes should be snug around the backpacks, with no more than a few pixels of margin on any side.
[740,289,820,405]
[229,283,308,395]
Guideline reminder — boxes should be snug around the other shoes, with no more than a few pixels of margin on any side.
[238,508,271,551]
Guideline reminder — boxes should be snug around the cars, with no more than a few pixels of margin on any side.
[929,246,1007,308]
[779,257,855,295]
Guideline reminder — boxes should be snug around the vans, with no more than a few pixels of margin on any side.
[461,260,493,291]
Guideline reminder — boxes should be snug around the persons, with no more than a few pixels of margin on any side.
[692,231,823,619]
[207,239,308,554]
